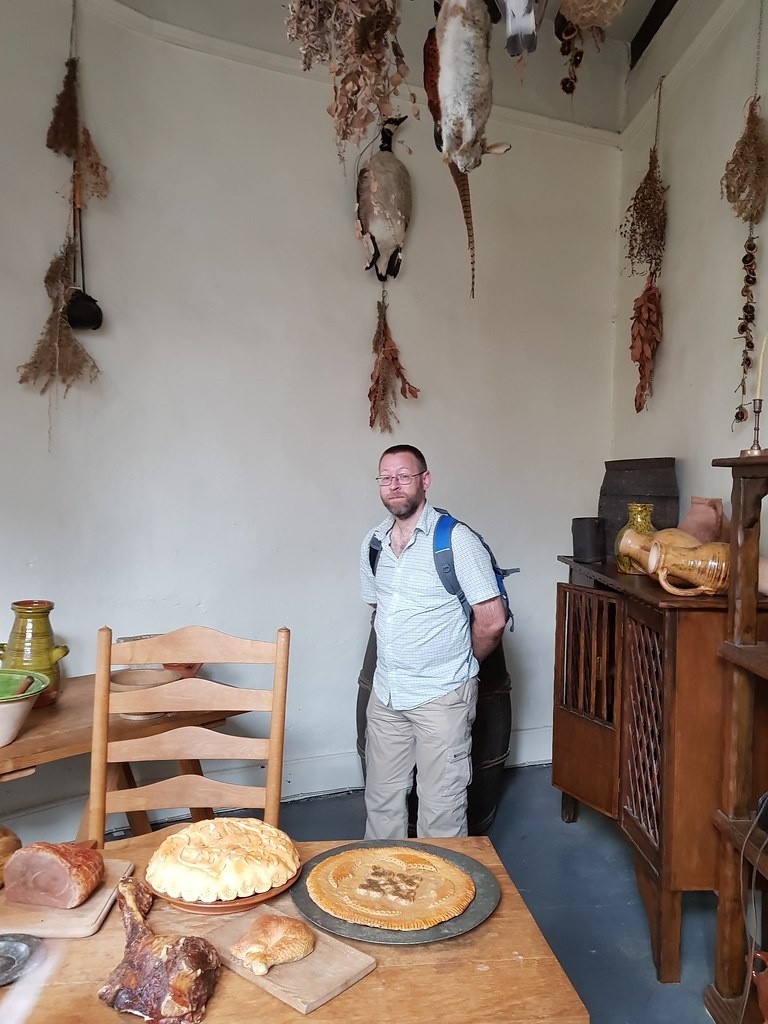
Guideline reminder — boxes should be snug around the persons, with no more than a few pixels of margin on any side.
[359,445,506,841]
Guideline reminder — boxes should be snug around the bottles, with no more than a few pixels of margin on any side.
[678,495,731,543]
[648,541,730,596]
[615,504,658,574]
[2,600,70,708]
[620,528,703,584]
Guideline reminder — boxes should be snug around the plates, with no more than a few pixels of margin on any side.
[142,860,300,915]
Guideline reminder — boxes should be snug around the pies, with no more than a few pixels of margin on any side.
[306,846,474,931]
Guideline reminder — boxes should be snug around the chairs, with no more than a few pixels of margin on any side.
[88,627,292,849]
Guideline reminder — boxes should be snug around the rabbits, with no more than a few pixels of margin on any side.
[435,0,512,174]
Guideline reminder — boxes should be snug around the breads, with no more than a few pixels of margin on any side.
[145,818,301,903]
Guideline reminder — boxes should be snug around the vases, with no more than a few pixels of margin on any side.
[0,600,70,708]
[679,496,731,545]
[649,541,731,596]
[615,502,659,575]
[619,528,704,586]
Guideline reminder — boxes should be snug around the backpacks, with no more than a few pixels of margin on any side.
[368,508,519,632]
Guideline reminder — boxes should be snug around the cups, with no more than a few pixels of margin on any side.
[572,517,606,563]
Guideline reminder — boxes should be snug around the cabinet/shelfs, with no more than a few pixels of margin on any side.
[551,455,768,1024]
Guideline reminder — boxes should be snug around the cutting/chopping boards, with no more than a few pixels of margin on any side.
[0,857,134,939]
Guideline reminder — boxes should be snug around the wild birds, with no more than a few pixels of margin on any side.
[357,115,412,282]
[422,2,476,299]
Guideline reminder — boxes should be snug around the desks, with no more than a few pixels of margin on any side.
[0,667,256,838]
[0,836,593,1022]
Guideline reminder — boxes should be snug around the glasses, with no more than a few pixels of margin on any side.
[375,471,425,486]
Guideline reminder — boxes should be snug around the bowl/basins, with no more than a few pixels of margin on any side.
[109,669,182,720]
[0,669,50,747]
